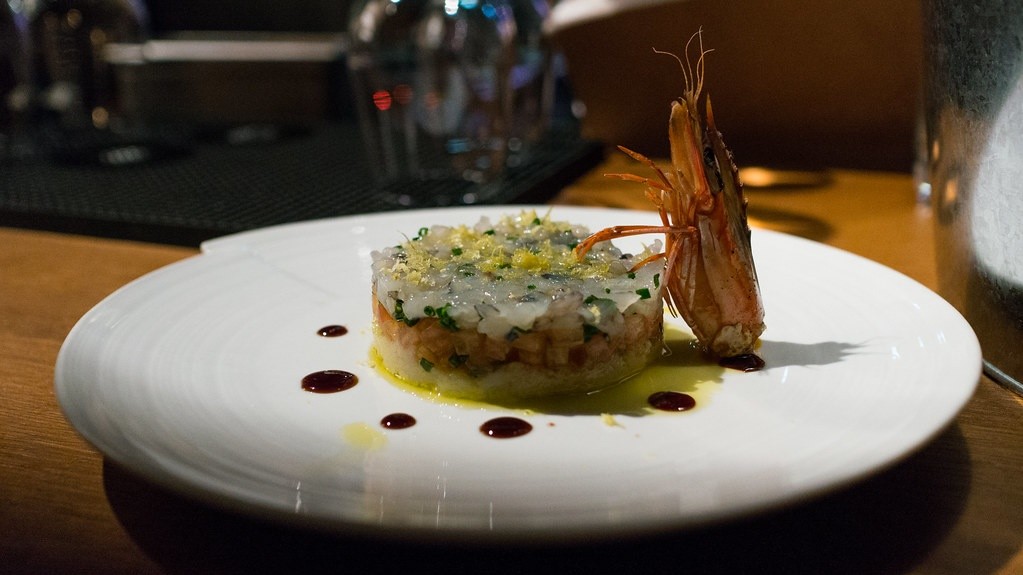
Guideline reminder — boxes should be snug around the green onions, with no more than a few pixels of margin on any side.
[395,218,659,373]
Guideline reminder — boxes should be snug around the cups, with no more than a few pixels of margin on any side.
[920,0,1023,392]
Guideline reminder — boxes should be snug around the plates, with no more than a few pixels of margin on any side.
[53,205,984,537]
